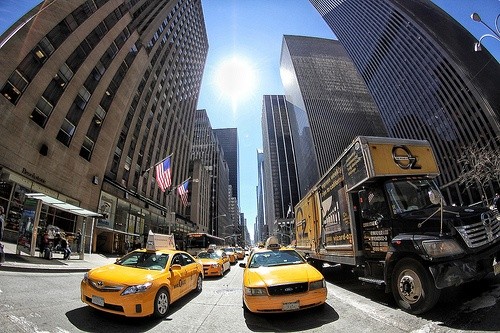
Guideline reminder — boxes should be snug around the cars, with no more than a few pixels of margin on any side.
[239,235,328,316]
[193,244,247,279]
[81,229,205,322]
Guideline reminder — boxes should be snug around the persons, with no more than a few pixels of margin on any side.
[23,217,71,260]
[407,196,426,210]
[0,206,4,240]
[76,229,82,253]
[124,238,141,254]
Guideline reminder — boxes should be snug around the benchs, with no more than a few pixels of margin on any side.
[16,235,79,257]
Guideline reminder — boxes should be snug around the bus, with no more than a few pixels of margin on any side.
[186,233,225,256]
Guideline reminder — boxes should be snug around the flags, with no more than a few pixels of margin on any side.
[156,158,171,192]
[178,181,189,207]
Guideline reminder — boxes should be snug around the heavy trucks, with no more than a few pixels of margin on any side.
[294,134,500,315]
